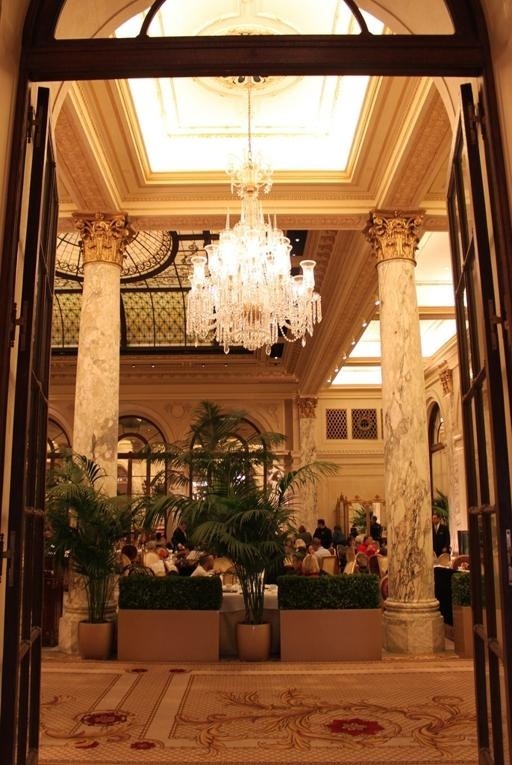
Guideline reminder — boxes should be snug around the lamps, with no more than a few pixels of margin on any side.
[187,28,321,357]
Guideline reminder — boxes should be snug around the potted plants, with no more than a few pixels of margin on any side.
[451,573,473,660]
[45,400,382,663]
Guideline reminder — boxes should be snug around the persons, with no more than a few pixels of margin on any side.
[432,512,451,558]
[120,513,388,582]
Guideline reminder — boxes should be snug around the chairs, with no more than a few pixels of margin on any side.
[322,541,471,597]
